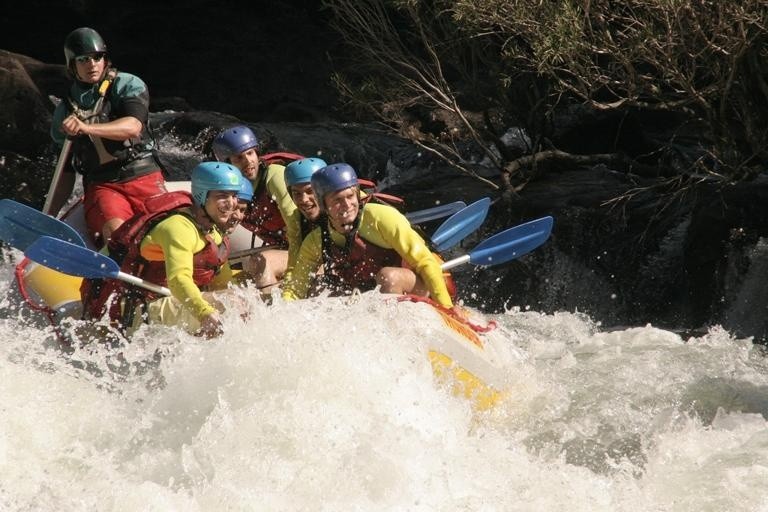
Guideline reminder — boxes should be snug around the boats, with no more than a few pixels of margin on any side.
[12,174,511,421]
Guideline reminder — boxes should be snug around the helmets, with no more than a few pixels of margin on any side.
[63,27,107,69]
[191,126,259,207]
[284,158,357,211]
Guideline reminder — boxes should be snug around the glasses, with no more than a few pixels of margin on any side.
[74,53,103,64]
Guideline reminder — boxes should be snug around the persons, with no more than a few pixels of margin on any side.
[214,125,298,296]
[280,158,468,326]
[51,28,170,250]
[79,161,254,337]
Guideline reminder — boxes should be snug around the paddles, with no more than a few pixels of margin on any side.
[430,197,490,253]
[23,236,171,297]
[226,201,467,260]
[441,216,554,272]
[0,199,86,252]
